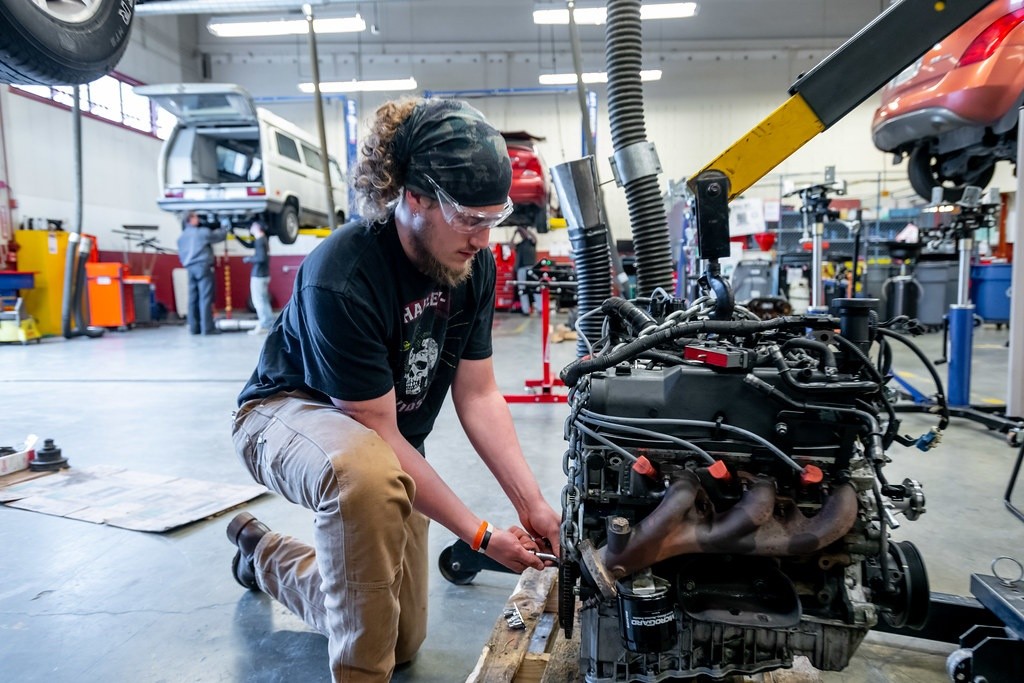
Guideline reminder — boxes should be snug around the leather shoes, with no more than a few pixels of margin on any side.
[226,511,271,589]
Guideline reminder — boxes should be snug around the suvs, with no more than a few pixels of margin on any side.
[486,127,565,234]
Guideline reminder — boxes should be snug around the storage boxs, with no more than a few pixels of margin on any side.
[867,257,1012,327]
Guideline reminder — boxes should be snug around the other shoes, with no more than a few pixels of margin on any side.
[201,329,222,334]
[247,326,268,335]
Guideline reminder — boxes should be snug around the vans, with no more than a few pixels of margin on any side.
[130,83,351,243]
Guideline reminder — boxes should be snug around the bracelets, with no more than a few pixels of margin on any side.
[472,521,493,554]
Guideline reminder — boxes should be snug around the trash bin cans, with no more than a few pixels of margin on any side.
[969,262,1012,322]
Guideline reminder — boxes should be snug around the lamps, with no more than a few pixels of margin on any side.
[120,0,702,94]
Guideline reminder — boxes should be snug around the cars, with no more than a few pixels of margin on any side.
[871,1,1023,205]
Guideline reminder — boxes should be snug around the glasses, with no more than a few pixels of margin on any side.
[420,172,514,234]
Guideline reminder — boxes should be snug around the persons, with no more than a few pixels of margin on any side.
[177,213,229,335]
[511,227,543,316]
[235,220,277,335]
[227,96,561,683]
